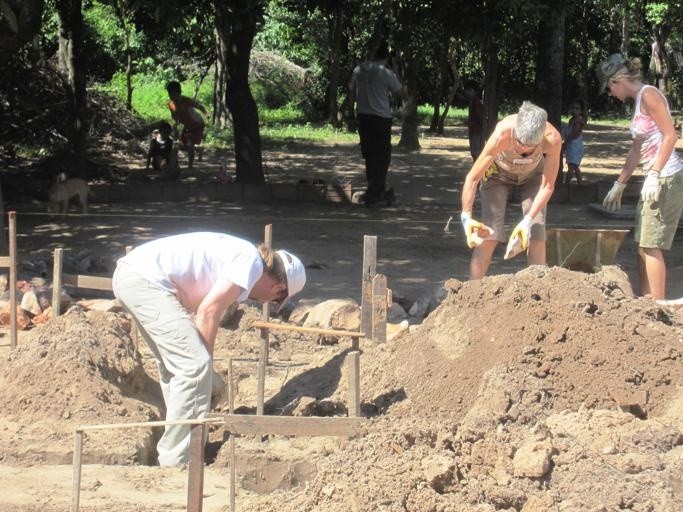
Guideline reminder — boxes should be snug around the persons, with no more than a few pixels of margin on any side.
[165,79,213,167]
[561,98,586,185]
[460,100,561,281]
[462,77,486,161]
[595,51,682,300]
[111,230,308,470]
[345,38,408,206]
[145,122,177,170]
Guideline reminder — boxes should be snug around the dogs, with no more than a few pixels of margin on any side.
[47,167,97,219]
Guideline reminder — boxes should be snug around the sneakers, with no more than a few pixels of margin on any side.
[368,198,387,208]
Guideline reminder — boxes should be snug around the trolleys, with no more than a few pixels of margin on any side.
[540,223,630,275]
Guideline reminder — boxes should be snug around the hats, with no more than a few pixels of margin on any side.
[595,53,626,96]
[274,250,306,313]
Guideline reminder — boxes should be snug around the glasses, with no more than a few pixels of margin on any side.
[605,81,616,93]
[514,134,540,147]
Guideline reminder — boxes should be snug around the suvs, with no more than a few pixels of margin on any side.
[154,60,192,80]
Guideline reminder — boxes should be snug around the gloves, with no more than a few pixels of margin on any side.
[602,181,627,212]
[509,215,537,251]
[460,212,495,249]
[640,169,660,202]
